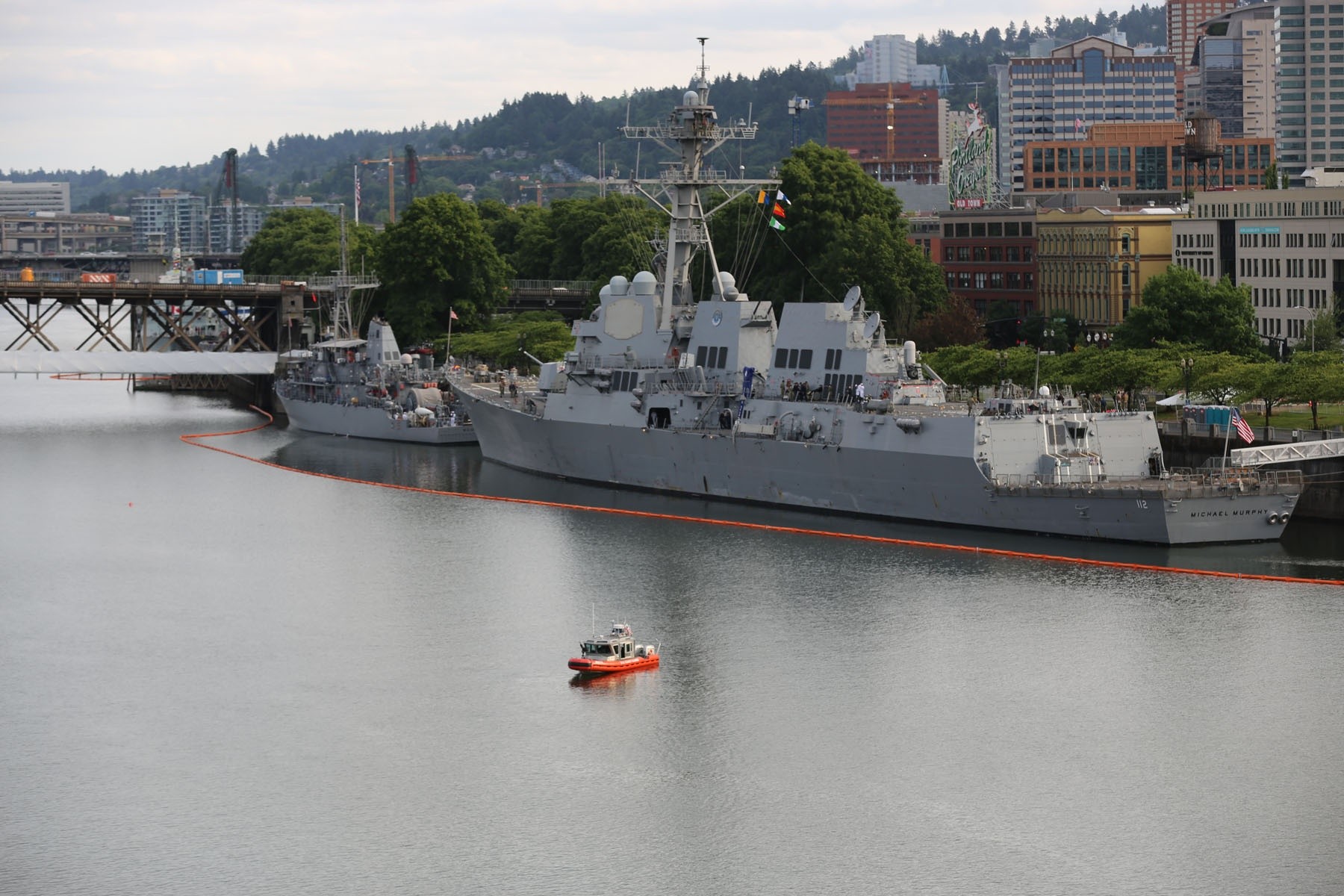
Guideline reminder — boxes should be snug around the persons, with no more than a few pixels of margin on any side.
[982,458,992,480]
[846,383,865,405]
[719,411,728,429]
[1056,393,1064,404]
[817,384,823,400]
[967,395,976,417]
[980,406,1006,416]
[1116,391,1146,412]
[780,379,810,403]
[499,379,518,397]
[1082,393,1107,412]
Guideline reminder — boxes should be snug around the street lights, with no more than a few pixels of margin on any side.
[1294,306,1314,352]
[1180,356,1193,406]
[1043,329,1054,355]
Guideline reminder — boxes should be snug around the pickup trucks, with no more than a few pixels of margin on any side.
[100,250,119,255]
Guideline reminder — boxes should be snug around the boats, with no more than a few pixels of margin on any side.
[141,305,258,352]
[568,602,661,674]
[272,202,477,445]
[446,38,1304,546]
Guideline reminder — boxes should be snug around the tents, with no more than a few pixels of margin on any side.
[1155,391,1234,421]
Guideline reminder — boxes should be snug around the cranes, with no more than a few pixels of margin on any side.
[519,182,599,208]
[361,149,474,226]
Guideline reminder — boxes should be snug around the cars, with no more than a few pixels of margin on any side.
[81,252,97,256]
[42,251,57,256]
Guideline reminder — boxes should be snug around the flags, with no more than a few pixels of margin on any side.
[451,310,459,321]
[776,189,791,205]
[1231,406,1255,444]
[758,190,771,205]
[773,202,785,218]
[769,216,785,231]
